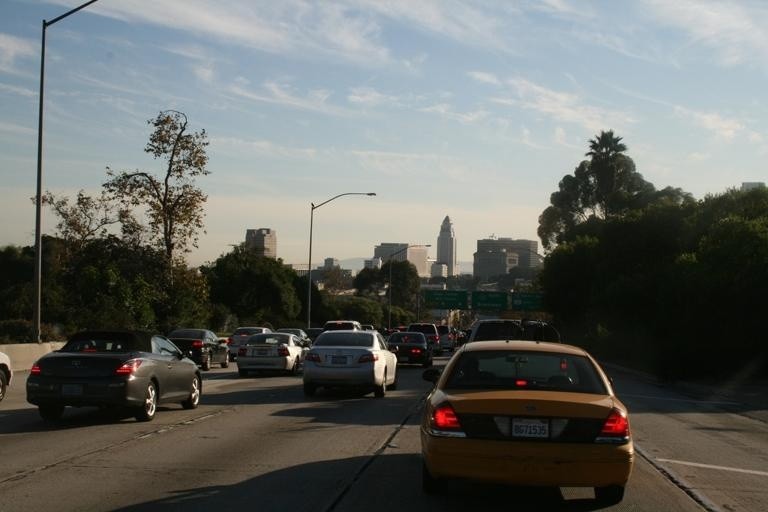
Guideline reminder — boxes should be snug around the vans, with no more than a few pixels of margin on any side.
[436,325,455,352]
[408,321,441,358]
[321,320,361,336]
[468,320,565,345]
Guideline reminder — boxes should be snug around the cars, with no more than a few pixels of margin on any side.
[237,333,308,377]
[1,352,13,400]
[25,328,202,423]
[303,329,397,399]
[422,341,633,503]
[451,328,472,347]
[383,331,433,369]
[277,329,312,349]
[227,327,274,362]
[166,327,230,370]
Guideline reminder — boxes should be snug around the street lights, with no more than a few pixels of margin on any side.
[307,192,377,328]
[33,0,98,344]
[388,245,431,329]
